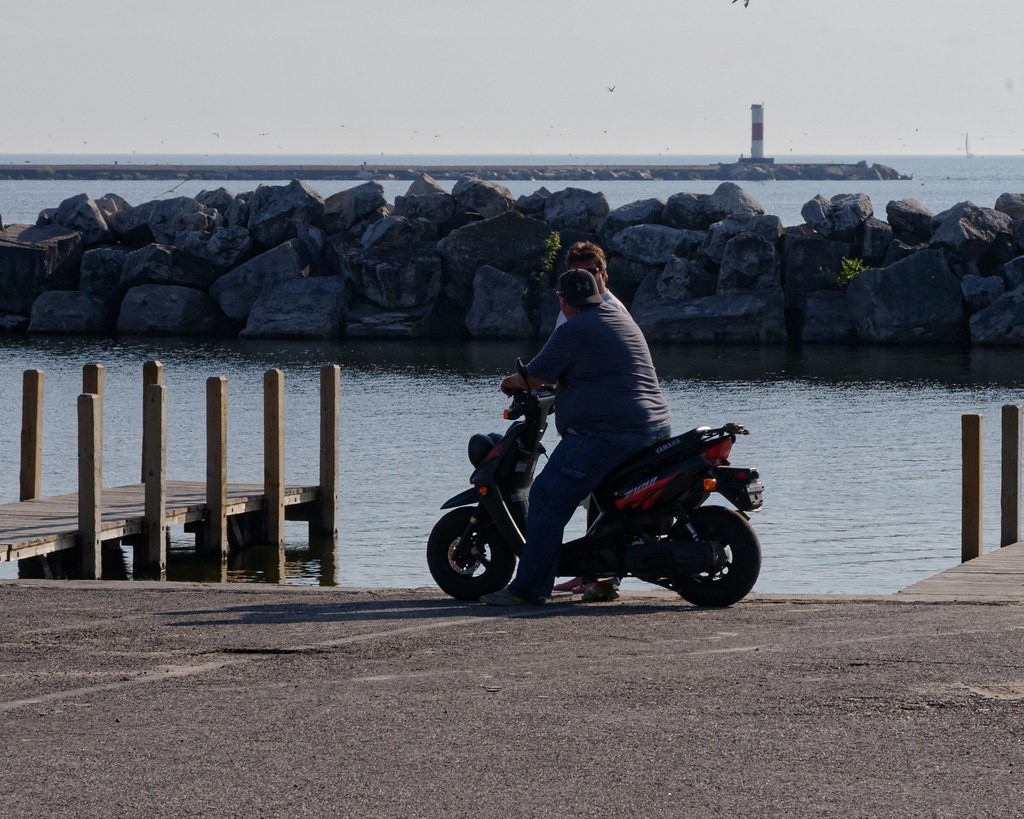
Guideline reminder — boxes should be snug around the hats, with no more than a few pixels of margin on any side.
[558,269,603,307]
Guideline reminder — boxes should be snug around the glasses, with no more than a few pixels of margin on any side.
[587,267,599,276]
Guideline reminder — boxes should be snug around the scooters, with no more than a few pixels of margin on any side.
[425,357,765,608]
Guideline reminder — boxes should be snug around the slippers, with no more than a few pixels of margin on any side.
[480,590,545,606]
[581,586,619,600]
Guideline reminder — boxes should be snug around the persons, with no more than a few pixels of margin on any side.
[479,242,671,601]
